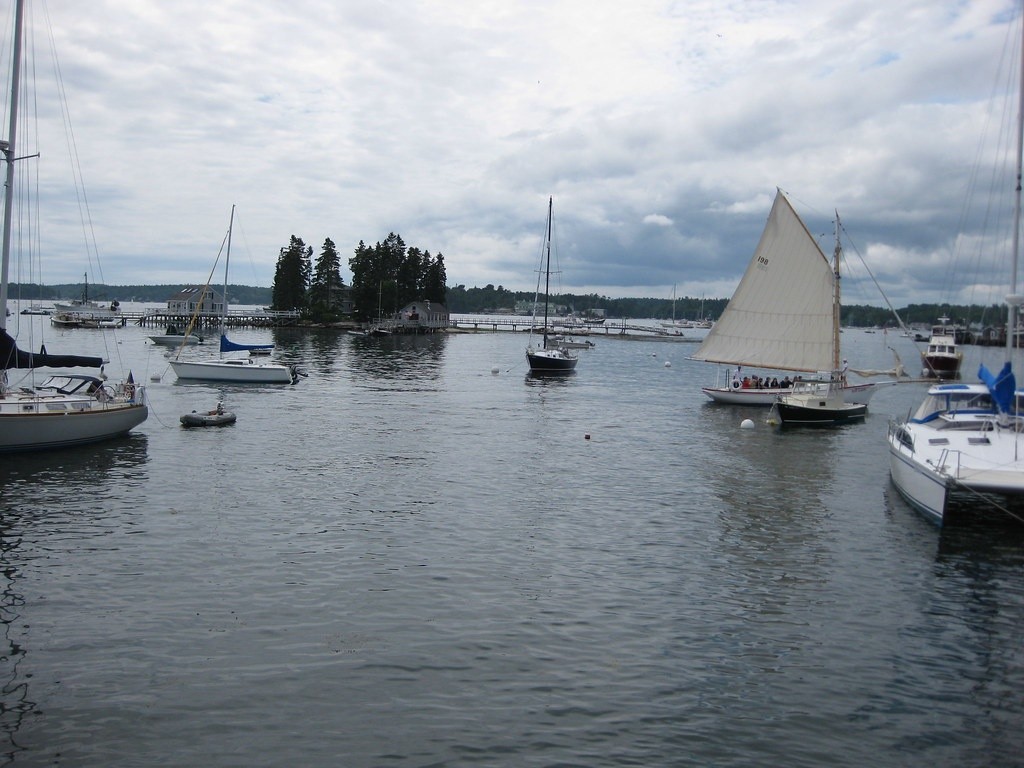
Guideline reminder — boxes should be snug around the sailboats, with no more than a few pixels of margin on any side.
[522,192,581,373]
[371,281,393,337]
[886,0,1024,532]
[166,202,297,385]
[659,283,714,329]
[678,186,869,427]
[0,0,154,452]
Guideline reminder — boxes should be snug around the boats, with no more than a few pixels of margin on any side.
[180,400,236,427]
[561,311,606,328]
[148,322,205,345]
[545,333,596,348]
[700,362,832,406]
[920,313,964,380]
[249,347,273,355]
[20,271,123,329]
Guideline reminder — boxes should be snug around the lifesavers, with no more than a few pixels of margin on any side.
[730,379,743,391]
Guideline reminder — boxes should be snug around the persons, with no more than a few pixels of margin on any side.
[742,375,802,389]
[732,366,742,382]
[841,358,848,387]
